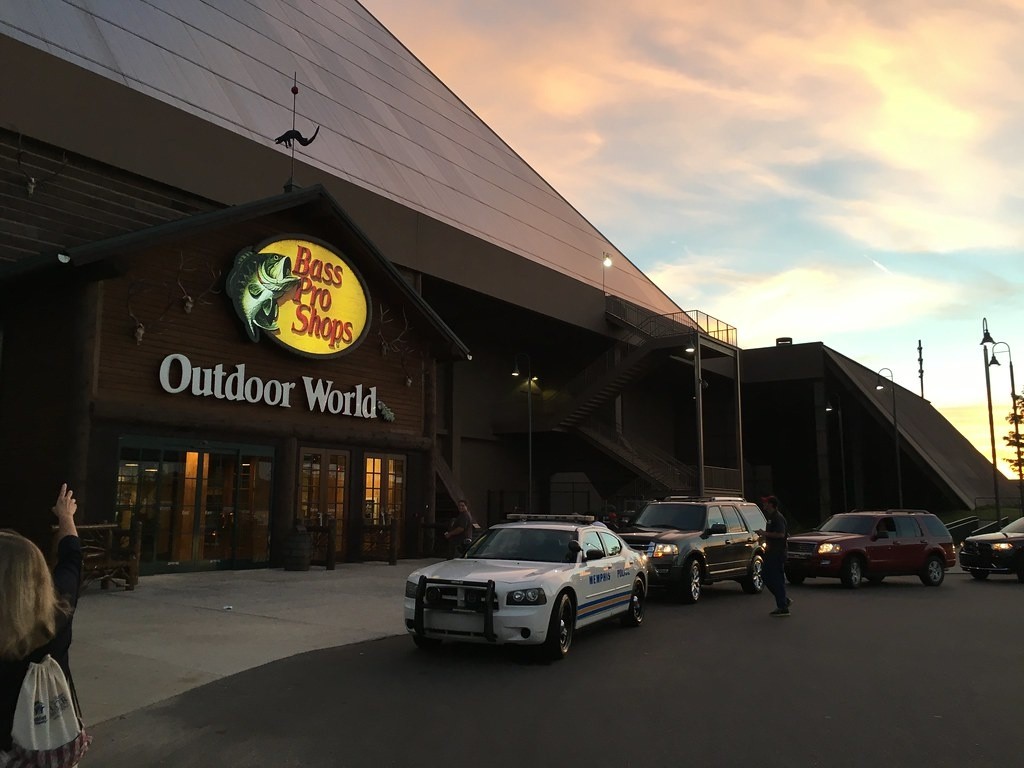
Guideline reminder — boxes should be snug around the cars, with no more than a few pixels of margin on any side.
[402,512,651,660]
[957,516,1024,583]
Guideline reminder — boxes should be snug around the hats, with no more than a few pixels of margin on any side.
[761,495,777,506]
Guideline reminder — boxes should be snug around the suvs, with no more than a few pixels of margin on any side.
[785,508,957,590]
[613,496,772,603]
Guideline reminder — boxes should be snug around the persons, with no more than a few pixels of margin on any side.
[755,496,793,617]
[444,499,472,560]
[0,483,92,768]
[543,533,573,562]
[608,505,616,519]
[875,520,888,533]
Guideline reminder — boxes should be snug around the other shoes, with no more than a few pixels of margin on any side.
[784,598,795,607]
[770,608,790,617]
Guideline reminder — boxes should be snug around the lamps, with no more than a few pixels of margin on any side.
[603,251,612,266]
[685,325,696,352]
[698,378,708,388]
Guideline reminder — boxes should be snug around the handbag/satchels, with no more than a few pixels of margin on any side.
[10,653,92,768]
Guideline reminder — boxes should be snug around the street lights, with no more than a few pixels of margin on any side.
[825,390,848,513]
[509,351,534,512]
[978,317,1024,521]
[874,367,904,508]
[683,325,702,496]
